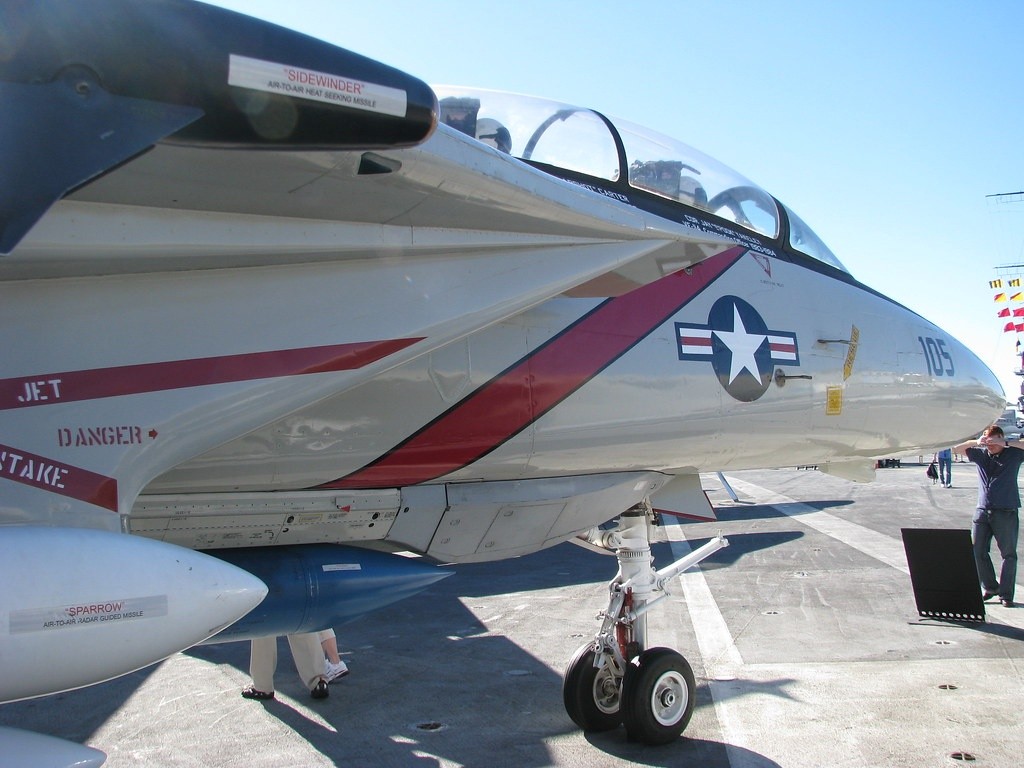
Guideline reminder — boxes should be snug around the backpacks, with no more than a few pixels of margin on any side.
[927,463,938,479]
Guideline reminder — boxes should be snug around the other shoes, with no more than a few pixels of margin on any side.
[946,484,952,488]
[325,659,349,682]
[310,679,329,699]
[983,585,999,601]
[941,484,945,488]
[1001,599,1015,607]
[242,686,274,700]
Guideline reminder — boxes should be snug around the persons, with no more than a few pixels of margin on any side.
[933,447,951,488]
[950,425,1024,608]
[240,625,349,700]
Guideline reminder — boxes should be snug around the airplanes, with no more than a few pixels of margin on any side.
[0,0,1011,768]
